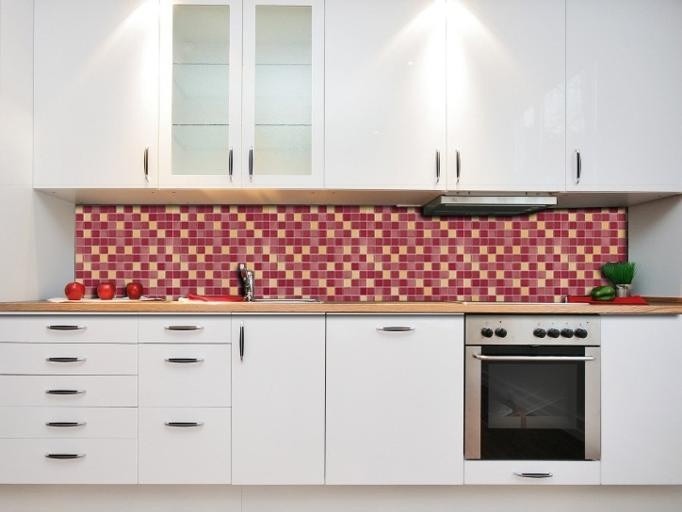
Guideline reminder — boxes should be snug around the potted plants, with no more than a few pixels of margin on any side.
[603,262,637,298]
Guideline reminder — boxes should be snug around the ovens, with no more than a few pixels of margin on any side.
[463,344,601,460]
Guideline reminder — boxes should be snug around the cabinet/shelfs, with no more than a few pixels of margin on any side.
[233,311,326,486]
[325,314,465,485]
[34,1,158,205]
[137,315,233,484]
[1,312,138,486]
[326,0,565,207]
[565,1,682,211]
[465,459,601,486]
[158,0,325,205]
[600,315,682,485]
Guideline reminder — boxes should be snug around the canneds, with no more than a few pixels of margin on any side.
[243,271,254,302]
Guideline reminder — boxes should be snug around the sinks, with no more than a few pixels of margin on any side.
[177,294,325,305]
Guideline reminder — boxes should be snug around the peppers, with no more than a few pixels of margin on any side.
[591,286,616,301]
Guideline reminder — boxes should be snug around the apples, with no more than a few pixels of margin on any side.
[126,283,143,299]
[97,283,114,300]
[65,282,85,300]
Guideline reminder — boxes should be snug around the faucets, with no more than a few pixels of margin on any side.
[238,263,254,302]
